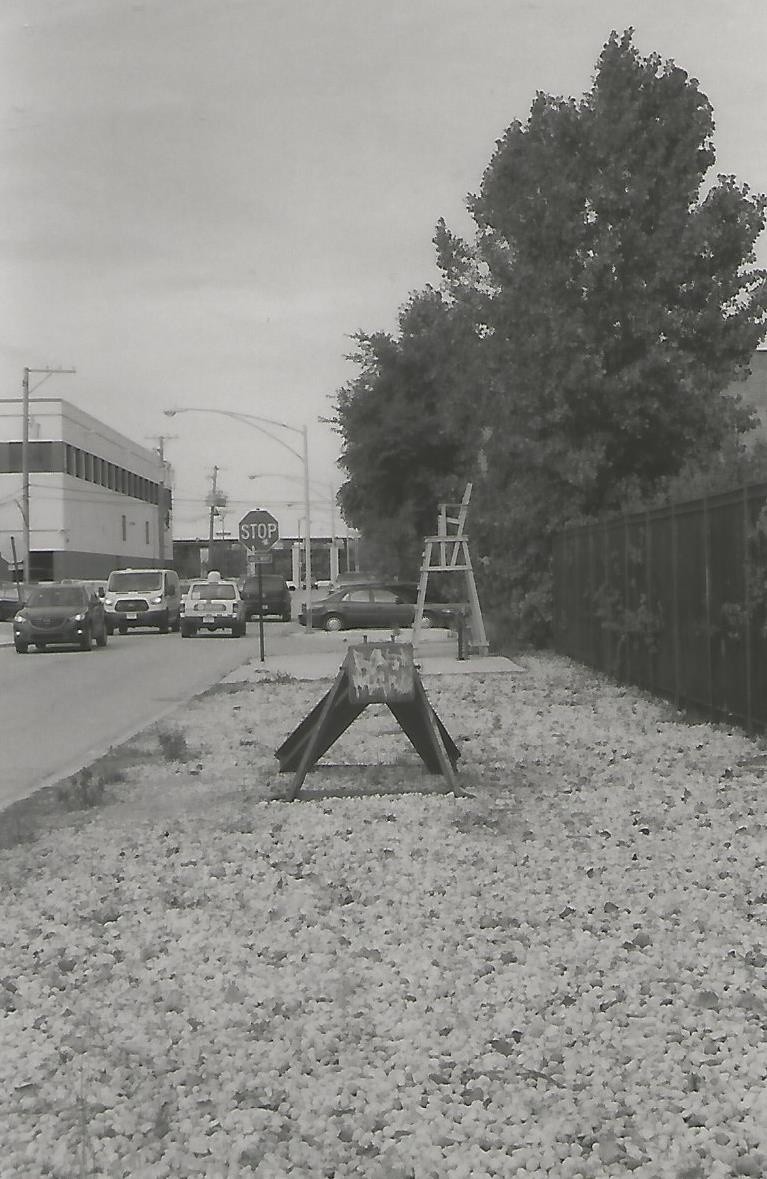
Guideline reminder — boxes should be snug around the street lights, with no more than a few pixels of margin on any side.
[164,406,312,632]
[288,503,351,572]
[247,473,340,581]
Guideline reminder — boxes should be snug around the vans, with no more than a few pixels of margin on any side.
[98,567,181,634]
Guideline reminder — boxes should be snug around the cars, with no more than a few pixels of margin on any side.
[297,583,462,629]
[13,583,109,653]
[285,573,377,594]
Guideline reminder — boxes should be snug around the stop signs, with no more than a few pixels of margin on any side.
[238,510,279,554]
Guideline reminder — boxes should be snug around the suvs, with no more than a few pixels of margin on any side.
[180,573,247,638]
[241,573,291,622]
